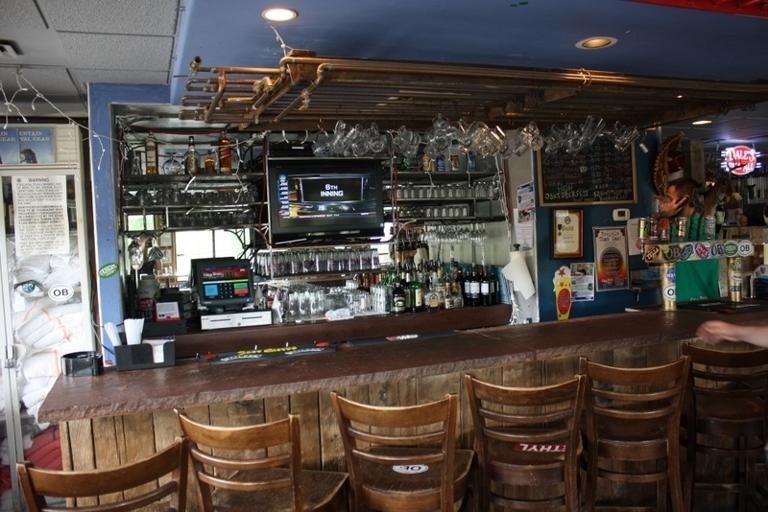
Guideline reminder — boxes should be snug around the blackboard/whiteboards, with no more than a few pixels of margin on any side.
[536,134,639,208]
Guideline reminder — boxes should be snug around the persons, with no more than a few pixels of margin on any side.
[15,301,84,428]
[694,318,768,350]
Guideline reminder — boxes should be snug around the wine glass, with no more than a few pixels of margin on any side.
[162,150,182,176]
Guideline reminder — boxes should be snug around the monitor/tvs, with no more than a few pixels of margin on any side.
[195,258,255,312]
[265,157,384,249]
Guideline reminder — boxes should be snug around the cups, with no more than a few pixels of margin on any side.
[308,113,646,161]
[121,184,257,229]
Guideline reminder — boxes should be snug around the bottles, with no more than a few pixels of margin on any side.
[422,152,432,174]
[257,180,502,325]
[205,150,216,175]
[184,135,201,175]
[435,155,446,172]
[145,129,160,176]
[449,154,460,172]
[217,130,233,176]
